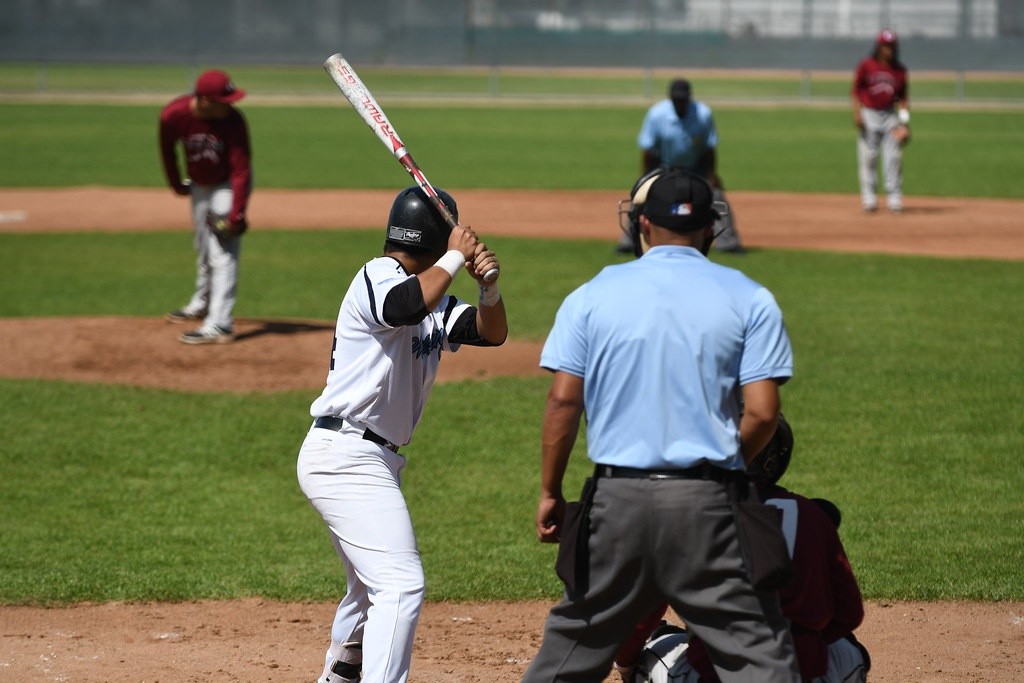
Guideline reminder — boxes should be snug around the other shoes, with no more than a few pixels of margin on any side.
[618,243,635,254]
[719,245,746,256]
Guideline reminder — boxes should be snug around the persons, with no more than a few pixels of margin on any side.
[614,77,751,254]
[520,164,793,683]
[614,407,872,683]
[851,29,911,215]
[159,70,253,346]
[297,186,509,683]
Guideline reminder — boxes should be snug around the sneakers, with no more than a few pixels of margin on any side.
[166,308,201,324]
[178,321,233,344]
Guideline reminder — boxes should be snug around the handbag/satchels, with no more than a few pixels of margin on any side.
[553,472,599,595]
[721,469,793,589]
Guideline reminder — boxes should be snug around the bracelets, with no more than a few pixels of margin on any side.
[433,249,467,278]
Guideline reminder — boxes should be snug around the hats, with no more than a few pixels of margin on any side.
[195,70,245,103]
[630,167,710,231]
[879,31,897,45]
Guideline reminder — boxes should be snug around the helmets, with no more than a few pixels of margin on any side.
[385,186,458,253]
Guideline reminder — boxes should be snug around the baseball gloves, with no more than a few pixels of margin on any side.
[206,208,247,248]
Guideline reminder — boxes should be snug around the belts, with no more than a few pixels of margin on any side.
[595,462,727,483]
[313,417,400,454]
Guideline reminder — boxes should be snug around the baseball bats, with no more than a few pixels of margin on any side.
[323,53,499,282]
